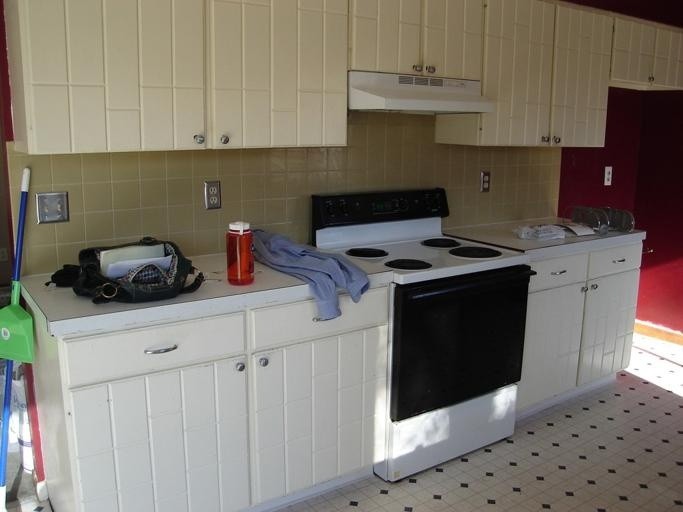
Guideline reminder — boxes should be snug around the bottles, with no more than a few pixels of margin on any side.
[227,220,255,285]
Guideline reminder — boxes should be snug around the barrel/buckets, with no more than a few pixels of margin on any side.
[6,370,35,476]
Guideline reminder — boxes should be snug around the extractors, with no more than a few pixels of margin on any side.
[348,69,497,117]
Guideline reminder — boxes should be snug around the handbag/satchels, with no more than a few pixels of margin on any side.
[71,237,191,303]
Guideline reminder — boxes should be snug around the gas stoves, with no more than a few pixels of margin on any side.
[327,230,532,286]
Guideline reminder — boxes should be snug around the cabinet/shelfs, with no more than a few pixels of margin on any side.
[351,1,484,84]
[16,284,390,512]
[2,2,350,159]
[515,239,642,412]
[608,12,682,93]
[435,0,615,150]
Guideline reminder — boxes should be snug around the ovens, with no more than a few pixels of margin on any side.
[373,264,535,482]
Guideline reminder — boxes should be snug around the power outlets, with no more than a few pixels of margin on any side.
[479,170,491,193]
[202,178,222,212]
